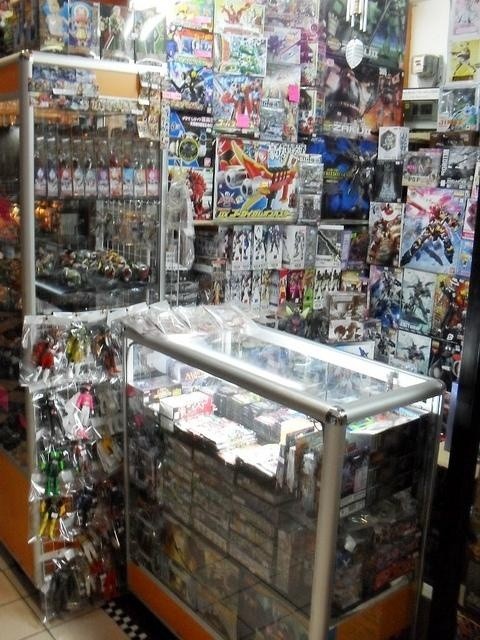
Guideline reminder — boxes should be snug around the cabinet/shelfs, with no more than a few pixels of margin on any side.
[0,48,169,622]
[119,317,446,640]
